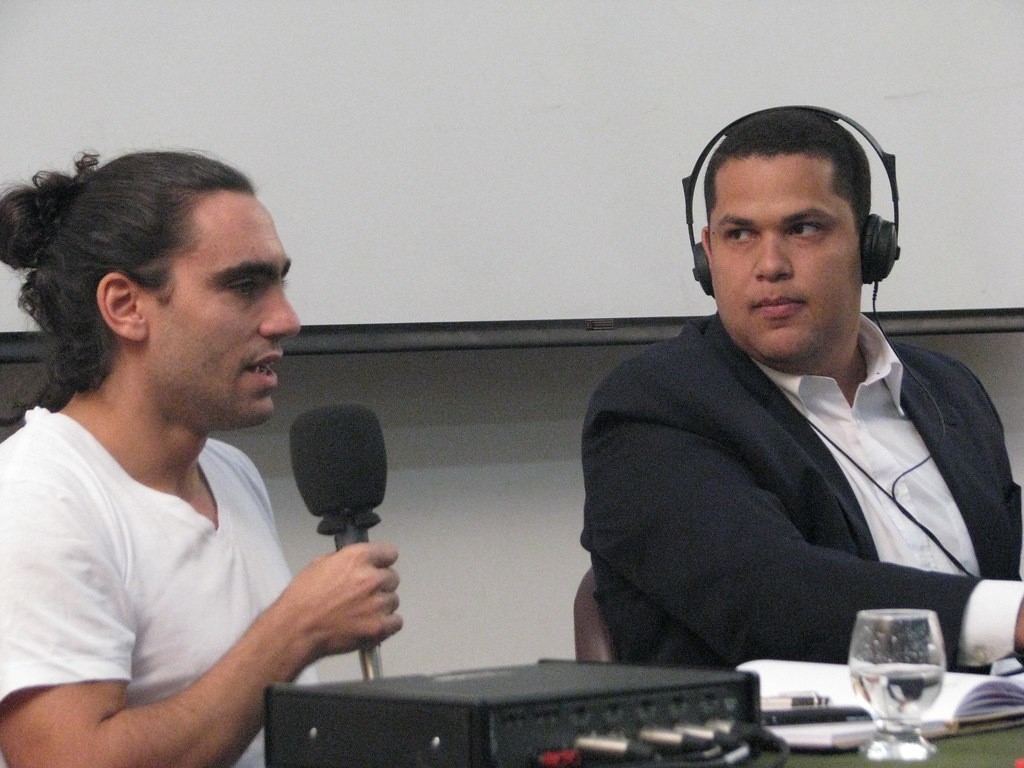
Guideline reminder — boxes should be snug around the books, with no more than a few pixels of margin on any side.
[726,655,1024,755]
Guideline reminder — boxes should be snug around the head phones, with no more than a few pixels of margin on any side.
[683,104,901,297]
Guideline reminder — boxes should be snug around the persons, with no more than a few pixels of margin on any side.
[0,151,404,768]
[576,102,1023,679]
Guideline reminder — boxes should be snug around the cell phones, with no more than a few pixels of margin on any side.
[761,696,866,724]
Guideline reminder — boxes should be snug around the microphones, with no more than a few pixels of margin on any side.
[290,404,387,682]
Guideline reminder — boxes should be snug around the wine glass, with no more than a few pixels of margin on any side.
[846,608,950,761]
